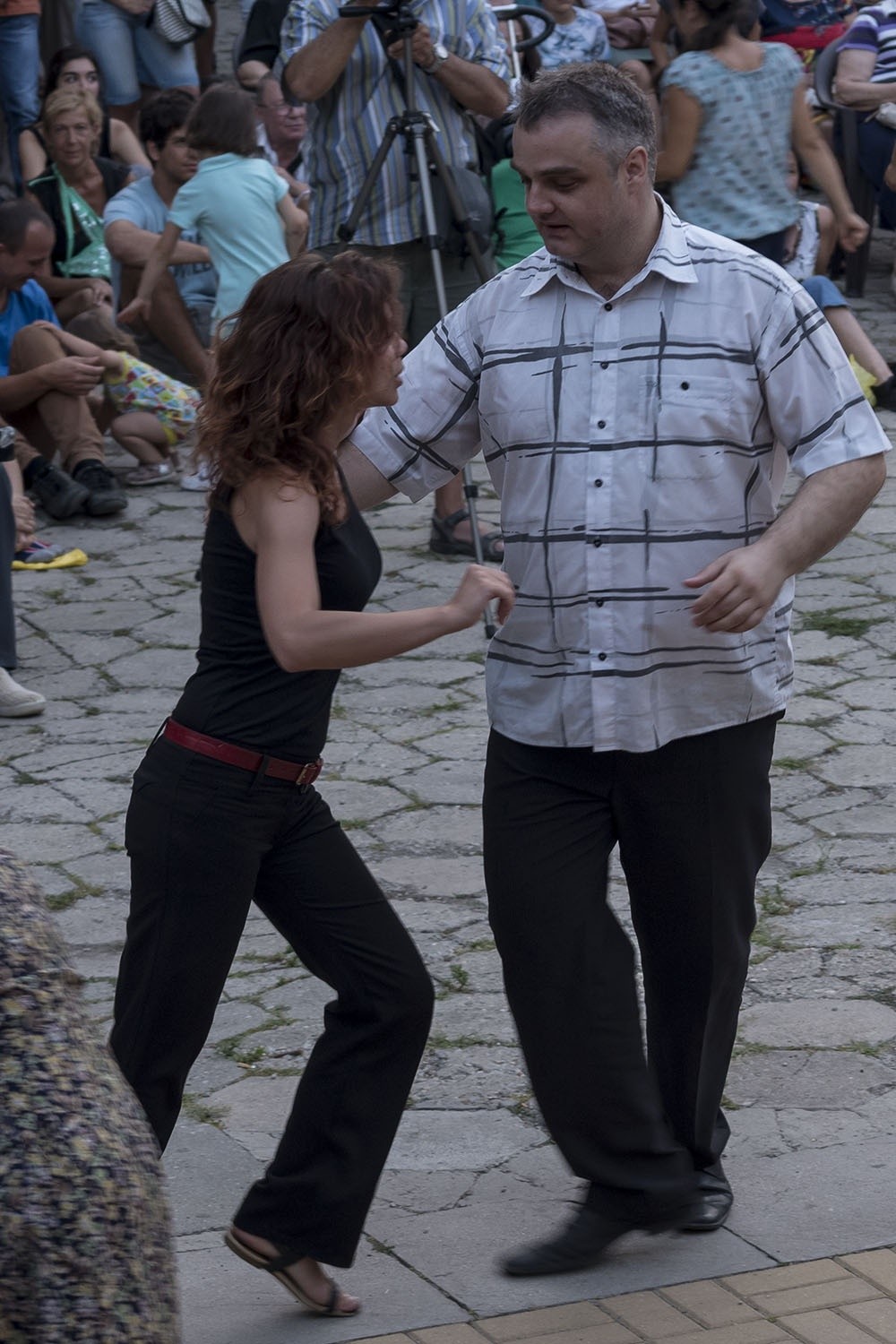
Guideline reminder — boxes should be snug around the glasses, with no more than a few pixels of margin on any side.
[256,103,306,116]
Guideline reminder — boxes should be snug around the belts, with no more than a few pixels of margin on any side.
[162,718,324,783]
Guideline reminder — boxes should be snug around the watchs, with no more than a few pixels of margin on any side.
[420,43,449,74]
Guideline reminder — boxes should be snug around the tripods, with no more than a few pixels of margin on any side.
[337,32,498,644]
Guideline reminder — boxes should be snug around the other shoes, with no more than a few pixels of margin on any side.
[29,460,91,519]
[870,375,896,413]
[179,461,212,492]
[77,462,129,514]
[126,457,176,485]
[0,666,45,715]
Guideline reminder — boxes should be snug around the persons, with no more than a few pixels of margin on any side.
[1,0,896,716]
[102,250,514,1317]
[339,54,893,1279]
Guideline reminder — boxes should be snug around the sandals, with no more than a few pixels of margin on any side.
[223,1222,360,1318]
[429,508,503,562]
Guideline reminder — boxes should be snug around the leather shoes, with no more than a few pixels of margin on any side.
[677,1158,733,1231]
[501,1182,707,1277]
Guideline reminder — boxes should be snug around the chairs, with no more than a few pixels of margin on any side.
[813,32,877,299]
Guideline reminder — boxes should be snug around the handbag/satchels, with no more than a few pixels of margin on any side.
[601,13,656,49]
[153,0,212,49]
[848,354,876,408]
[421,164,494,257]
